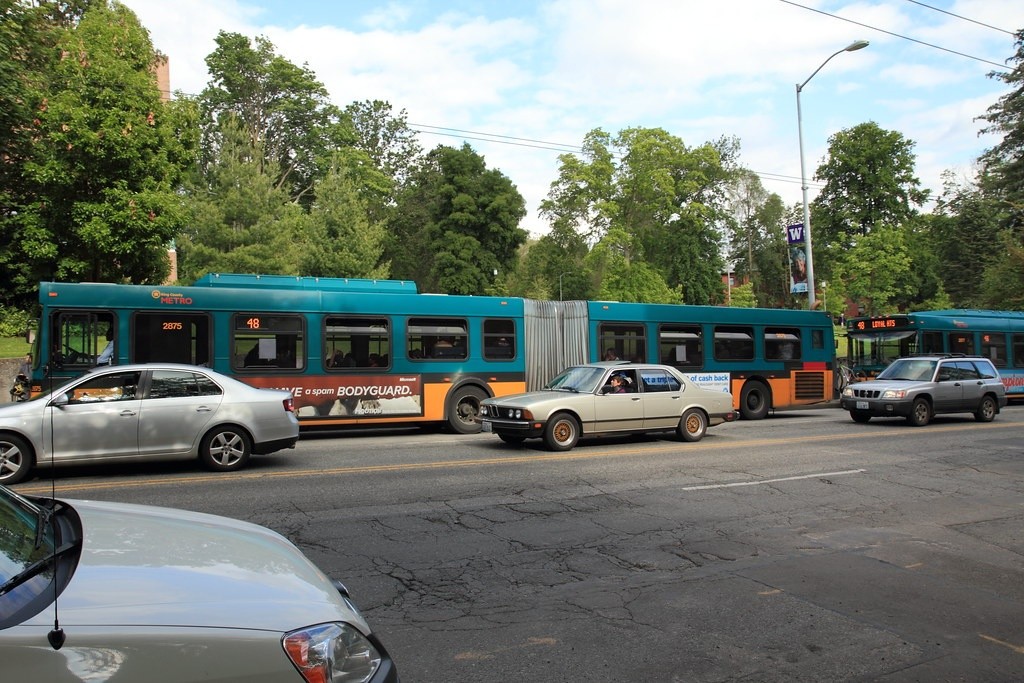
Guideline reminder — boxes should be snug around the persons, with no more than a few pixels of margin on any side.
[14,353,32,402]
[369,354,379,367]
[326,350,343,368]
[791,250,808,284]
[604,348,619,361]
[606,375,625,394]
[778,340,793,359]
[96,327,114,364]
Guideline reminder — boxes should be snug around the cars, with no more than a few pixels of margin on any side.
[474,360,740,452]
[0,479,399,683]
[0,363,301,486]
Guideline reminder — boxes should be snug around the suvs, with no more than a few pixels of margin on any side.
[840,352,1009,427]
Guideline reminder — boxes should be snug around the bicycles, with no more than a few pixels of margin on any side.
[836,357,868,392]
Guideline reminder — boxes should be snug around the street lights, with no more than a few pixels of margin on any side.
[560,272,572,301]
[796,40,870,306]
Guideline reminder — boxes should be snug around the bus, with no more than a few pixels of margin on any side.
[24,273,835,436]
[834,308,1024,399]
